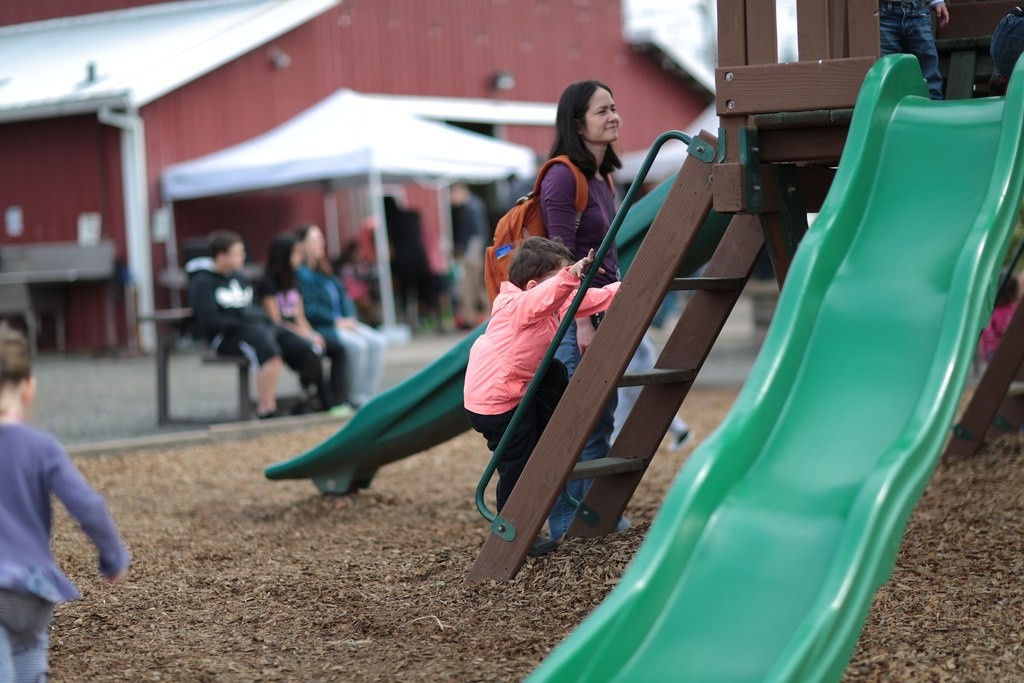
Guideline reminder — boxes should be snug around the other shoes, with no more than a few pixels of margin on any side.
[612,517,632,532]
[252,387,385,418]
[528,534,556,555]
[669,426,695,452]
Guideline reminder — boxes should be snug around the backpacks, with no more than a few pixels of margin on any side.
[484,154,615,312]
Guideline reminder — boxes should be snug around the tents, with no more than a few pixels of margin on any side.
[161,86,537,352]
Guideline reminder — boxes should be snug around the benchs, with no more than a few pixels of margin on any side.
[134,307,330,424]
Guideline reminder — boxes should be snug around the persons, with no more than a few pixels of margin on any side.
[184,221,381,420]
[0,321,132,683]
[383,181,489,337]
[540,80,632,541]
[975,272,1024,364]
[989,5,1024,97]
[609,333,695,452]
[878,0,950,100]
[462,236,622,557]
